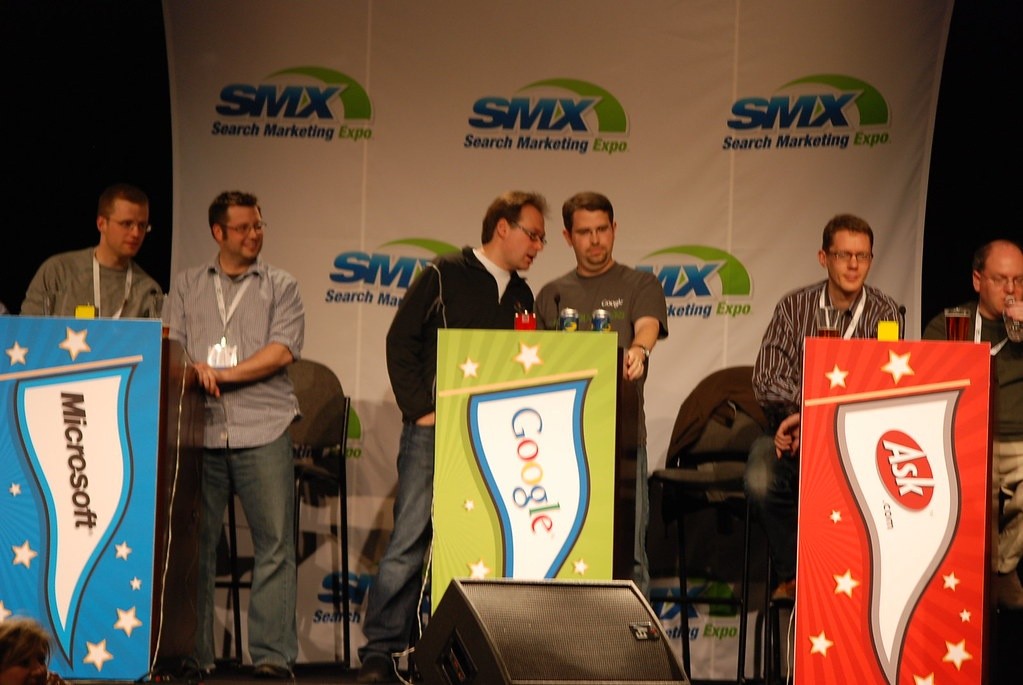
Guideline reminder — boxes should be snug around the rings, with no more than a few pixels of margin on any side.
[639,362,642,367]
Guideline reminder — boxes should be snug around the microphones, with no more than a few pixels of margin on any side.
[845,310,854,316]
[150,288,157,319]
[898,304,907,339]
[554,294,560,317]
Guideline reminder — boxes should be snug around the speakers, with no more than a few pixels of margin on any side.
[408,578,691,685]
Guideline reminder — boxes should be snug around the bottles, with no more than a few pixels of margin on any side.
[1003,295,1022,341]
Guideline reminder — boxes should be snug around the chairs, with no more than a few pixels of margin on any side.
[217,357,355,658]
[648,368,799,685]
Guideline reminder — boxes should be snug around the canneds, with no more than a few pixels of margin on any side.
[589,308,611,332]
[560,307,578,332]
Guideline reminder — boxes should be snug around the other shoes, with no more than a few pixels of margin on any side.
[254,664,287,677]
[357,653,394,685]
[998,570,1023,608]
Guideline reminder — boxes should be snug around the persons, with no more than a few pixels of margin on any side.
[0,617,65,685]
[746,212,900,600]
[532,191,669,598]
[356,190,548,685]
[20,185,164,318]
[169,190,307,685]
[923,240,1023,611]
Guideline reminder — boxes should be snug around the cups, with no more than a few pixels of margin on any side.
[591,310,610,332]
[945,307,971,340]
[559,307,578,331]
[147,292,172,338]
[816,308,843,337]
[43,289,63,316]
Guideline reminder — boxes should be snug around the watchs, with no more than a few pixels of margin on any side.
[635,344,649,358]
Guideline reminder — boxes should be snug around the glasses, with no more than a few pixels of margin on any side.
[105,215,152,232]
[507,216,548,249]
[822,249,874,263]
[217,221,269,236]
